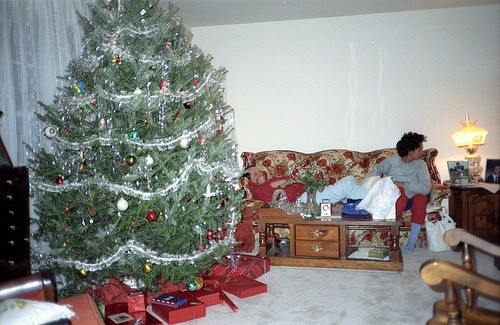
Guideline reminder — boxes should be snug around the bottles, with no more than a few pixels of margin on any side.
[320,198,331,216]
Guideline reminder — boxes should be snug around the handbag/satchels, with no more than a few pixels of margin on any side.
[425,206,456,253]
[354,178,402,220]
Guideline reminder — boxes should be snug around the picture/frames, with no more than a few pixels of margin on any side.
[485,159,500,184]
[447,160,470,184]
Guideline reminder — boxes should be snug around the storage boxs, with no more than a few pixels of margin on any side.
[105,255,271,325]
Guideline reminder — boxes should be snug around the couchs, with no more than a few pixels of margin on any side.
[241,148,451,248]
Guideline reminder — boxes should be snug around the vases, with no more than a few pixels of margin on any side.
[301,189,321,218]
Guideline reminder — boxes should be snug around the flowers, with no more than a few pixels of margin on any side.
[280,166,330,192]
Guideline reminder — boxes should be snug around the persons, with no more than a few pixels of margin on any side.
[454,162,464,176]
[367,132,431,253]
[487,164,500,183]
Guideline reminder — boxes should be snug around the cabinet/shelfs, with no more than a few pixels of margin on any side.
[447,185,500,249]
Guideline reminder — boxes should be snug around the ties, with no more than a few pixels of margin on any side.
[496,177,497,182]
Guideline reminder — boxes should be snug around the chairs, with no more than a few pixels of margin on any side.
[0,270,105,325]
[419,228,500,325]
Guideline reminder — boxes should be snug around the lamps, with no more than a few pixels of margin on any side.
[450,114,487,182]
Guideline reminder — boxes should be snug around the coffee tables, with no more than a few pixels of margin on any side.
[256,213,402,272]
